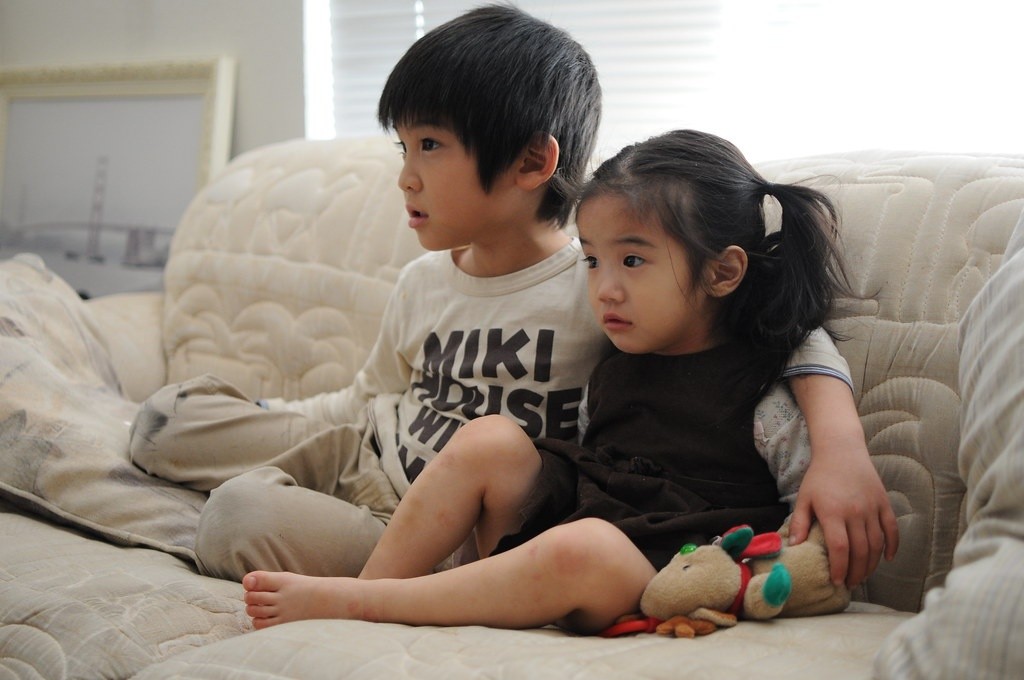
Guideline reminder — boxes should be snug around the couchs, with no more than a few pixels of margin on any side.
[0,138,1024,680]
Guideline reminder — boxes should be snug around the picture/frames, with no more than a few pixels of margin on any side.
[0,54,237,300]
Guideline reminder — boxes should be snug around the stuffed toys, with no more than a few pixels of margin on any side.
[606,511,852,639]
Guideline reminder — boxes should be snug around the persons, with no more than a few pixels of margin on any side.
[242,131,839,631]
[129,3,898,584]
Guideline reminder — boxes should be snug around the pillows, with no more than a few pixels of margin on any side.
[868,215,1024,680]
[0,251,209,575]
